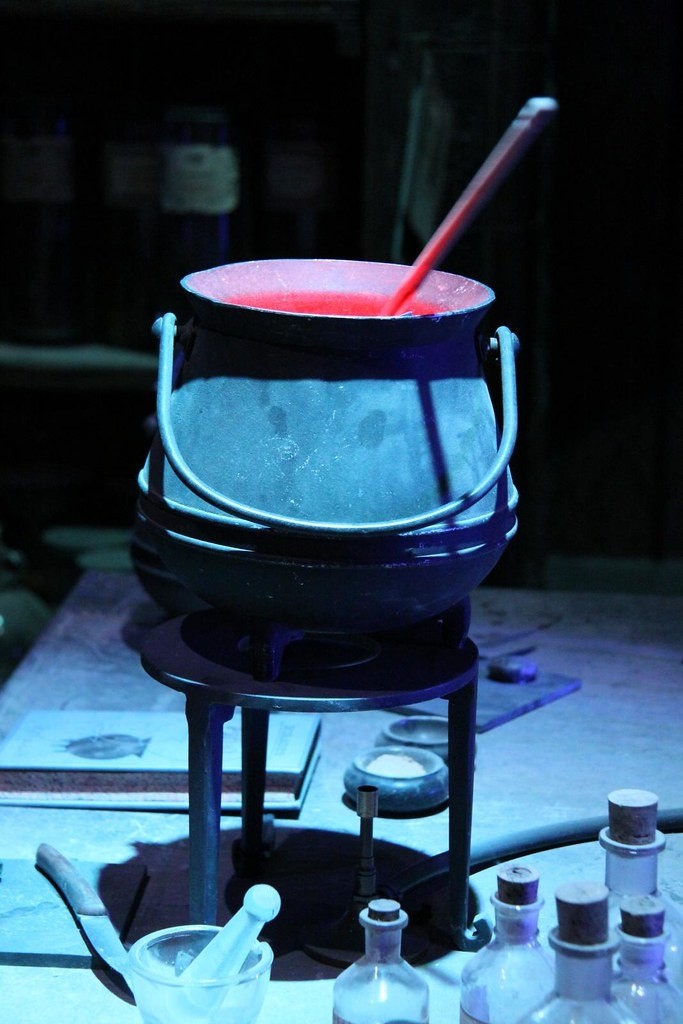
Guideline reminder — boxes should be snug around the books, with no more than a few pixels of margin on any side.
[0,708,324,819]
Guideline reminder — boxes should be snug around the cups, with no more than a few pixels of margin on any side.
[123,924,274,1023]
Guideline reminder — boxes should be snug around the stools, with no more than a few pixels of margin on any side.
[136,618,480,934]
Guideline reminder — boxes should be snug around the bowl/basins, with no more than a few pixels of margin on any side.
[374,715,449,762]
[344,745,449,812]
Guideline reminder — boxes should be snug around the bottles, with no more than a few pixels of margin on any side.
[332,900,429,1024]
[614,898,683,1024]
[460,865,555,1024]
[598,788,683,991]
[516,882,643,1024]
